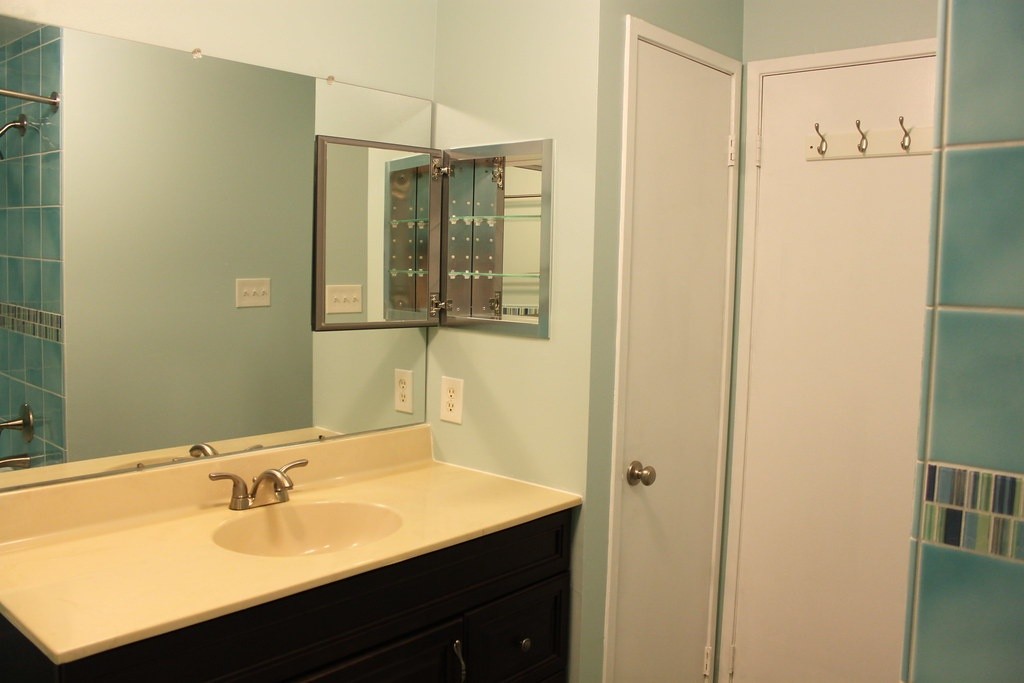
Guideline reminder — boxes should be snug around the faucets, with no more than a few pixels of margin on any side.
[251,471,293,497]
[187,444,217,458]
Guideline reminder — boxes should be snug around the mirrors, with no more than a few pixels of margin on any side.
[439,139,553,340]
[1,14,435,493]
[310,135,446,332]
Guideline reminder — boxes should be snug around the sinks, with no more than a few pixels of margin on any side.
[212,500,405,557]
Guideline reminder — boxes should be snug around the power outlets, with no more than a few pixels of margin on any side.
[440,376,463,424]
[394,368,413,414]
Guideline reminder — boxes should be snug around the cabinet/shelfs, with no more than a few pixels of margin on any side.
[0,505,583,683]
